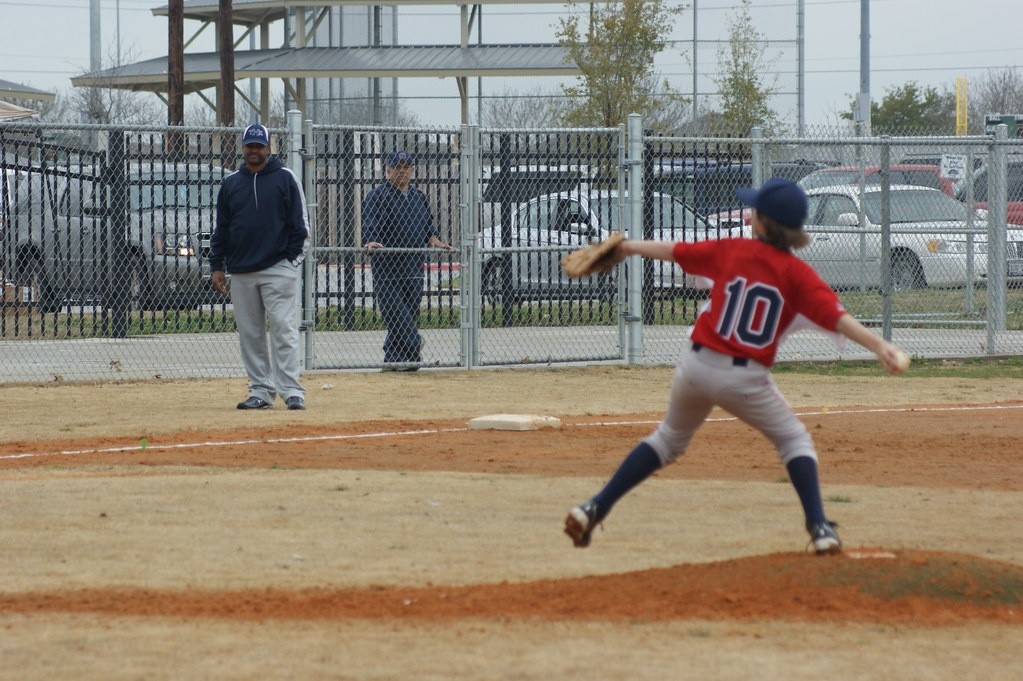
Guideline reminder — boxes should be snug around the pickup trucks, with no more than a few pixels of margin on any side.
[708,166,957,235]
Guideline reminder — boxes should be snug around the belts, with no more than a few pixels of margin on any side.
[692,343,746,366]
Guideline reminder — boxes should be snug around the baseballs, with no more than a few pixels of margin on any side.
[895,350,911,371]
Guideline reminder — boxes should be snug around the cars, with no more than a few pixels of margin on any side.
[0,162,234,311]
[706,184,1023,290]
[476,190,715,301]
[958,158,1023,228]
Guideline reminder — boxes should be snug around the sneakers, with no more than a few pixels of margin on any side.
[286,396,305,410]
[809,520,843,552]
[562,501,596,548]
[237,396,273,409]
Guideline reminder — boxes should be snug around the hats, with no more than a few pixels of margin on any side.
[387,150,414,166]
[242,123,270,146]
[736,177,809,229]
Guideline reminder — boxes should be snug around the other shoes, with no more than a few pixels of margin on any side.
[383,359,418,372]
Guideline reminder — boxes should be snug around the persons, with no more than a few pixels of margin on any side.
[209,125,311,409]
[360,151,453,372]
[566,177,908,555]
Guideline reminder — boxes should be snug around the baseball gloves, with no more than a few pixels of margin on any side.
[562,230,627,278]
